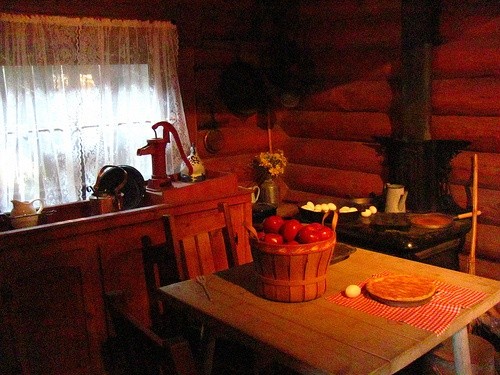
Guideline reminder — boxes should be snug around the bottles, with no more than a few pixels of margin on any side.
[179,146,206,182]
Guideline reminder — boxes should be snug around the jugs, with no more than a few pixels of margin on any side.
[239,185,260,204]
[9,199,45,228]
[88,164,127,217]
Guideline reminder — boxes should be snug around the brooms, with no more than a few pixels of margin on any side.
[464,154,500,334]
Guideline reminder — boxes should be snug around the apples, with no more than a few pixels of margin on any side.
[254,215,332,246]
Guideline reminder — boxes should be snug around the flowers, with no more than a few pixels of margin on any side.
[257,148,287,178]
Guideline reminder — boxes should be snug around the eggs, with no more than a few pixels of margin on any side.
[345,284,361,297]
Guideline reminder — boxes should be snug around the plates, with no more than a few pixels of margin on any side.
[330,242,356,266]
[366,276,434,303]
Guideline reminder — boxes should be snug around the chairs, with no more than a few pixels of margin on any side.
[104,291,198,375]
[162,202,239,282]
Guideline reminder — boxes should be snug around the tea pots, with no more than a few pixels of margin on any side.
[383,182,409,214]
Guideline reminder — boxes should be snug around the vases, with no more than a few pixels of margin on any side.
[259,174,281,209]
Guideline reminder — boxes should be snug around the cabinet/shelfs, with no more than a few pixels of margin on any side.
[0,186,255,375]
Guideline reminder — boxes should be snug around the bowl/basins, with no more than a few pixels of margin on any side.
[351,197,379,211]
[297,204,362,224]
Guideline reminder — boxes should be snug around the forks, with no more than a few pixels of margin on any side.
[196,275,213,302]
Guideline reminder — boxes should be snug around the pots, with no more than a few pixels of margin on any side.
[204,102,224,152]
[408,210,482,229]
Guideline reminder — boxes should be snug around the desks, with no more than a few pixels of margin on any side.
[157,244,500,375]
[282,201,471,271]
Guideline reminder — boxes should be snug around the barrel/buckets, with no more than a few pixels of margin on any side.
[242,209,337,303]
